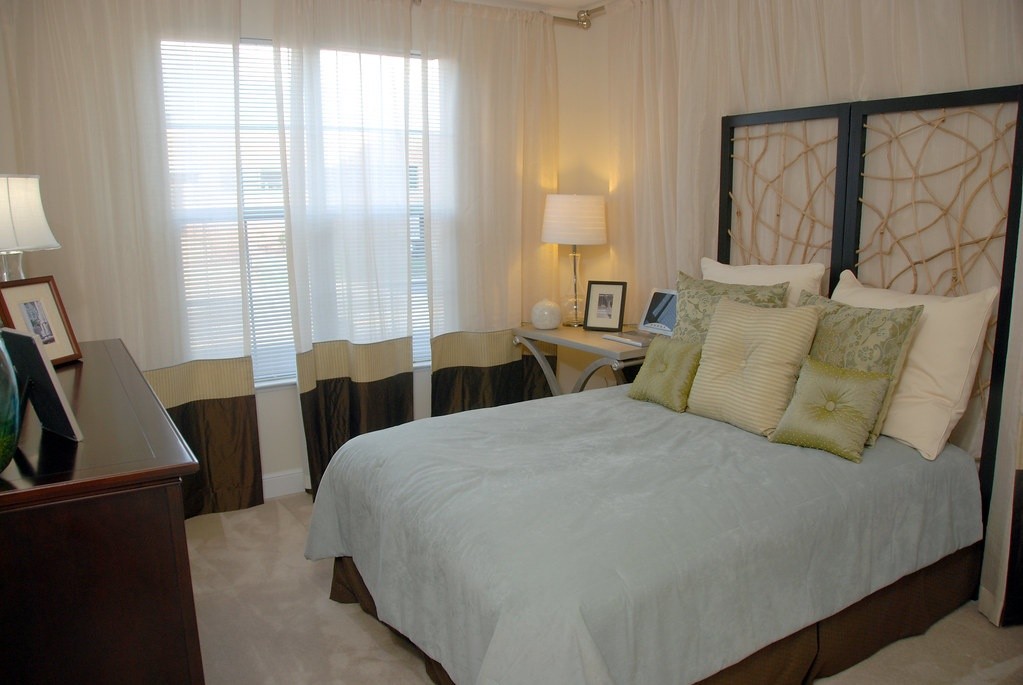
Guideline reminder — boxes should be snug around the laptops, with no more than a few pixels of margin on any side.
[602,288,679,347]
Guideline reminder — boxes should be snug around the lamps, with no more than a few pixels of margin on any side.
[0,173,63,281]
[540,192,607,326]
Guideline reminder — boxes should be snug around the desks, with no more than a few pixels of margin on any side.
[1,338,207,685]
[511,324,657,399]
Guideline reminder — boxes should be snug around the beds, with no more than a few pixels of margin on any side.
[308,84,1022,685]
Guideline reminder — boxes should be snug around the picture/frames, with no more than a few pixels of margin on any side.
[583,281,628,331]
[0,327,82,442]
[0,276,82,366]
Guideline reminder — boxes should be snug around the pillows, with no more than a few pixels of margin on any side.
[686,302,824,435]
[774,356,895,466]
[698,255,824,310]
[830,270,999,460]
[628,270,790,411]
[791,289,924,448]
